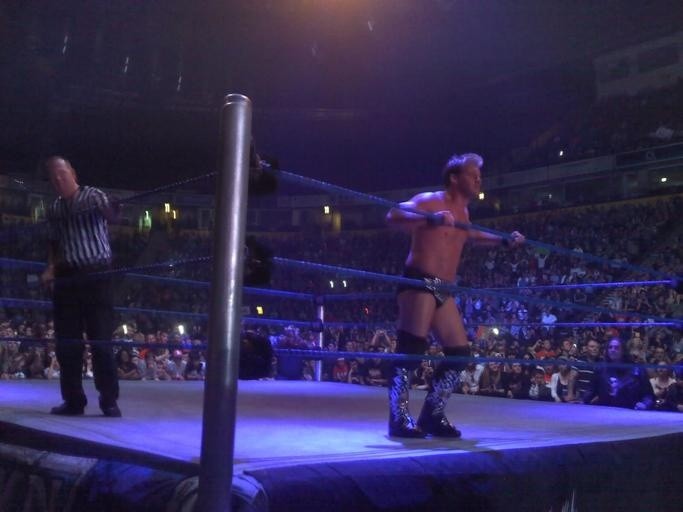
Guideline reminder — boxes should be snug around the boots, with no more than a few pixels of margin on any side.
[417,364,461,439]
[390,366,425,438]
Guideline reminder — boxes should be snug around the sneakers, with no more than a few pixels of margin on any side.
[51,402,84,416]
[99,395,121,417]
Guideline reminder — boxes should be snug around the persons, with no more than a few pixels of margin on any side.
[384,153,525,440]
[1,90,683,413]
[41,156,125,416]
